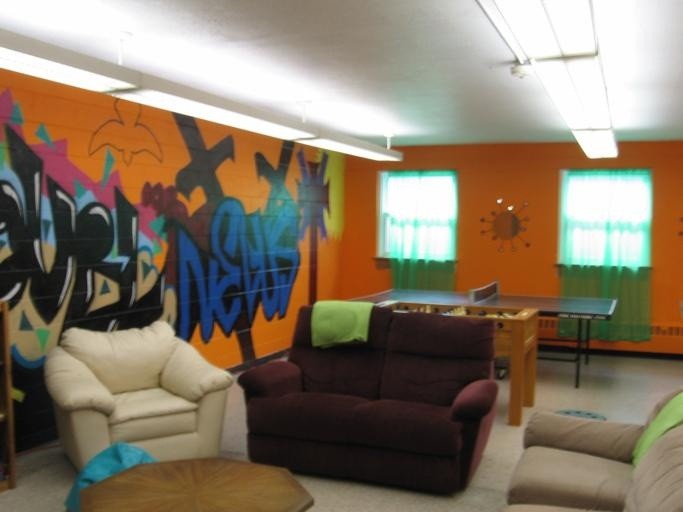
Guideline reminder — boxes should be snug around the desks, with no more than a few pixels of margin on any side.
[350,278,618,388]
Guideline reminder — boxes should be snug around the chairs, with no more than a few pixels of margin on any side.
[504,389,683,512]
[44,321,234,474]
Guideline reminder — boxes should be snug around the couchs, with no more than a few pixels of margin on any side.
[237,306,500,496]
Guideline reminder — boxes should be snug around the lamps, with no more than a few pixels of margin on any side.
[0,29,404,161]
[492,0,619,159]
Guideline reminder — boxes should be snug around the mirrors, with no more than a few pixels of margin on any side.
[479,197,532,253]
